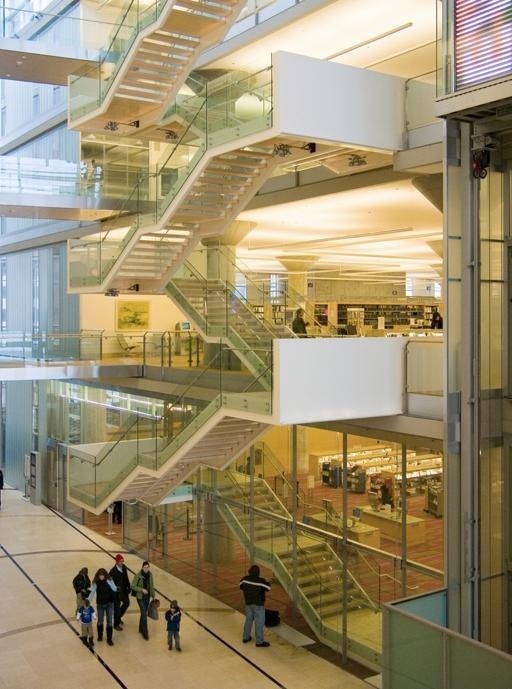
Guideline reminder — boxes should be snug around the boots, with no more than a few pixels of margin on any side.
[82,636,94,646]
[96,620,123,645]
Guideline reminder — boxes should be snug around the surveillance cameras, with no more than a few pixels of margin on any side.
[136,284,139,291]
[136,120,139,127]
[309,143,315,153]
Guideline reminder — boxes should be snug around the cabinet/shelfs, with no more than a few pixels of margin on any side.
[251,300,439,336]
[307,444,444,501]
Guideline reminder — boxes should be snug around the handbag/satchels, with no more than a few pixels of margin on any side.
[130,589,136,596]
[147,595,159,620]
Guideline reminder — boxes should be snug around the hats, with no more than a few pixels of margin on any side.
[116,553,124,564]
[169,599,176,607]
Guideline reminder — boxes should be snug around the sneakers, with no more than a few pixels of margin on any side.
[242,636,271,647]
[167,645,182,651]
[139,625,148,639]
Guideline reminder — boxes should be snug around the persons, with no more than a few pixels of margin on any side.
[292,308,310,338]
[164,600,183,651]
[112,501,122,524]
[430,312,443,329]
[72,554,155,646]
[239,565,272,647]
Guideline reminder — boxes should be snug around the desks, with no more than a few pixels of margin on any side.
[308,504,426,559]
